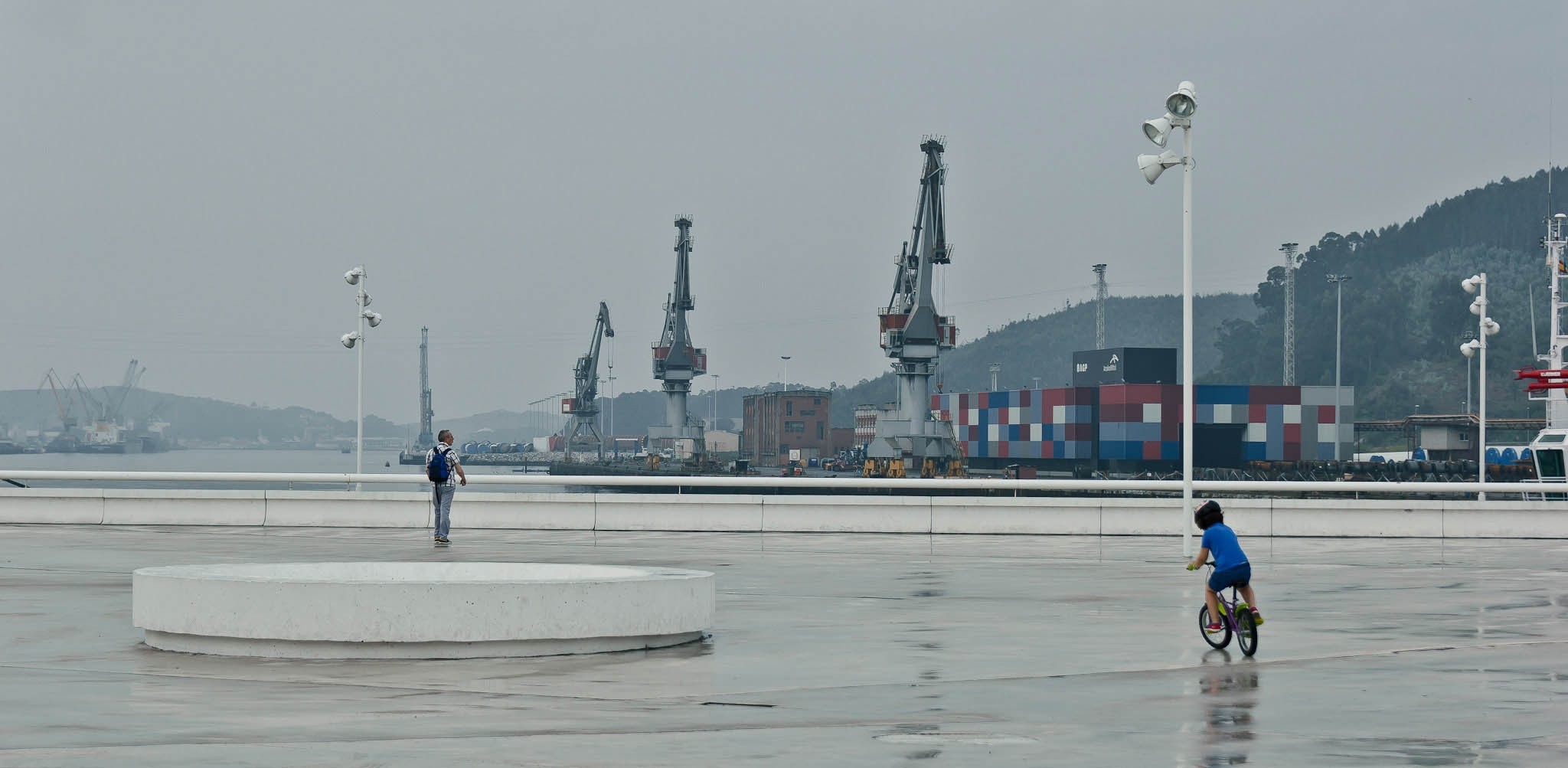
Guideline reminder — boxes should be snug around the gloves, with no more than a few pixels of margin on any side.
[1187,561,1198,571]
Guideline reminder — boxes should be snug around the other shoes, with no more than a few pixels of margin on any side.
[1251,607,1265,625]
[434,538,453,545]
[1203,623,1220,632]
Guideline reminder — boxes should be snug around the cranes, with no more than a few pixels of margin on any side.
[706,374,719,431]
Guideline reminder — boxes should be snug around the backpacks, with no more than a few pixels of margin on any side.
[428,447,453,482]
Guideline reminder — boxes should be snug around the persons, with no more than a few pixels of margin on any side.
[1187,500,1264,632]
[425,429,467,545]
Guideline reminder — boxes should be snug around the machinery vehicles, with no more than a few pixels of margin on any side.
[833,449,855,472]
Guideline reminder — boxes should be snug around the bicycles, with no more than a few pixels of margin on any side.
[1187,560,1258,656]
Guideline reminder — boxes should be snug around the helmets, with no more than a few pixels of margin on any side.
[1194,499,1224,529]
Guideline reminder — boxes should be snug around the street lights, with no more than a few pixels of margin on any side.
[606,377,616,436]
[341,264,381,488]
[1461,272,1500,498]
[1415,404,1421,447]
[1462,401,1466,413]
[1032,377,1042,390]
[1324,274,1353,463]
[556,393,567,436]
[1136,80,1199,557]
[599,380,606,435]
[568,391,573,429]
[781,357,791,392]
[1527,407,1529,441]
[545,396,556,435]
[529,399,545,436]
